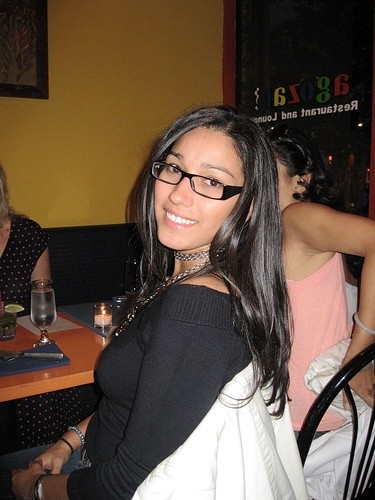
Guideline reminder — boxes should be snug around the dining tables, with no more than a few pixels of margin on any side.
[0,298,137,403]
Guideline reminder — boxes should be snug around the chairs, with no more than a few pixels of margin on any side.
[130,343,375,500]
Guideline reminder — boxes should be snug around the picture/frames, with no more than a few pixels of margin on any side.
[0,0,49,100]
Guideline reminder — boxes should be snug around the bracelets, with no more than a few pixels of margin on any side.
[60,437,74,454]
[68,427,85,446]
[34,474,53,500]
[354,313,375,335]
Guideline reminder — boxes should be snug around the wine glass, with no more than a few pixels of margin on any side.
[30,279,57,348]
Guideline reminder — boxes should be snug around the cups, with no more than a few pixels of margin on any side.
[94,303,113,328]
[0,301,17,341]
[113,296,128,326]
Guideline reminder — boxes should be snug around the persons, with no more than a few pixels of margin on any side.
[0,165,88,450]
[0,104,295,500]
[261,124,375,441]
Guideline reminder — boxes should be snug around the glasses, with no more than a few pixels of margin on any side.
[151,161,243,200]
[266,123,313,169]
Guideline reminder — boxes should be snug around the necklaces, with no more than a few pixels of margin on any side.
[110,262,208,337]
[173,250,210,261]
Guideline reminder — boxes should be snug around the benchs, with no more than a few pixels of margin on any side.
[0,220,176,454]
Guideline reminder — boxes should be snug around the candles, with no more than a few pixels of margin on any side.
[93,302,113,328]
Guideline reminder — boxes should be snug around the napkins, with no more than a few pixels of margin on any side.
[0,342,71,377]
[16,315,83,336]
